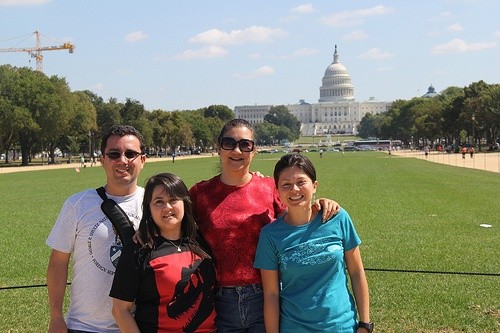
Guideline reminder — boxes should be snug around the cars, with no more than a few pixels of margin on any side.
[260,141,342,153]
[344,145,360,151]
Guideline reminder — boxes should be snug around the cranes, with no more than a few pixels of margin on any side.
[1,29,77,74]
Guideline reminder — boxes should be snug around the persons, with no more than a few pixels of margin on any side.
[45,125,147,333]
[133,119,341,333]
[253,153,370,333]
[48,139,499,167]
[109,173,222,333]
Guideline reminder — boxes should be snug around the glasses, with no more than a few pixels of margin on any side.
[219,137,254,151]
[103,151,142,159]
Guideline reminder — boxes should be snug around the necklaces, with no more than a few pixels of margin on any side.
[168,237,182,252]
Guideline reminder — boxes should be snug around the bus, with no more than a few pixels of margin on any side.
[353,139,392,150]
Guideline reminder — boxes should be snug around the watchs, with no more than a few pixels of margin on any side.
[358,321,375,333]
